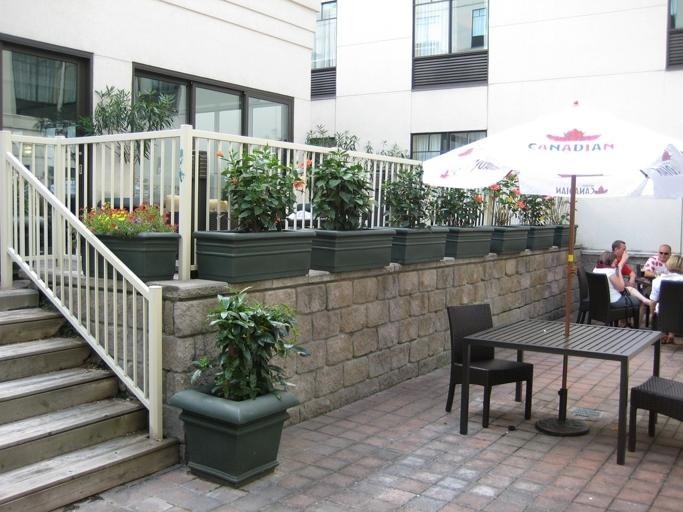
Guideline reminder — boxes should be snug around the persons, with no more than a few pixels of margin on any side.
[640,244,672,327]
[592,251,651,327]
[649,255,683,344]
[612,240,636,287]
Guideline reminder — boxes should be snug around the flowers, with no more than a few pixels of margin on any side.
[486,170,527,227]
[217,143,314,232]
[428,187,487,228]
[519,194,555,225]
[80,201,175,239]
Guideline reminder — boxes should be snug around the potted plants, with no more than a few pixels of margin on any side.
[381,165,451,265]
[309,147,396,272]
[554,196,578,248]
[166,285,311,487]
[192,231,316,284]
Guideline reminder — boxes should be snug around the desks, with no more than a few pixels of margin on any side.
[460,318,662,465]
[624,274,652,328]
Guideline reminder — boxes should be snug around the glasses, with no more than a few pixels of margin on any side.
[660,252,669,255]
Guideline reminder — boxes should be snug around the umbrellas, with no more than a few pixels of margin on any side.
[422,98,683,424]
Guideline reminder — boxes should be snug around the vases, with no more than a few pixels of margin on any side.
[491,226,530,254]
[528,226,556,250]
[445,226,495,259]
[81,230,181,282]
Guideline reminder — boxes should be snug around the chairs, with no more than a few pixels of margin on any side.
[652,280,683,337]
[577,266,590,323]
[586,272,639,327]
[628,375,683,451]
[445,303,533,428]
